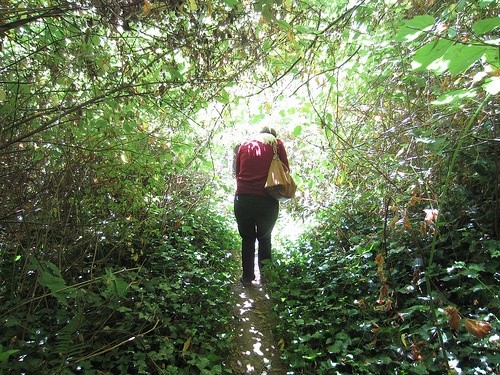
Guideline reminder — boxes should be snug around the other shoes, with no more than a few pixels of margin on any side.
[240,276,251,286]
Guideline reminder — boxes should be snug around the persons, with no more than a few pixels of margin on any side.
[234,125,292,287]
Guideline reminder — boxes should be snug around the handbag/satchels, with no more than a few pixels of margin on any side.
[264,138,297,204]
